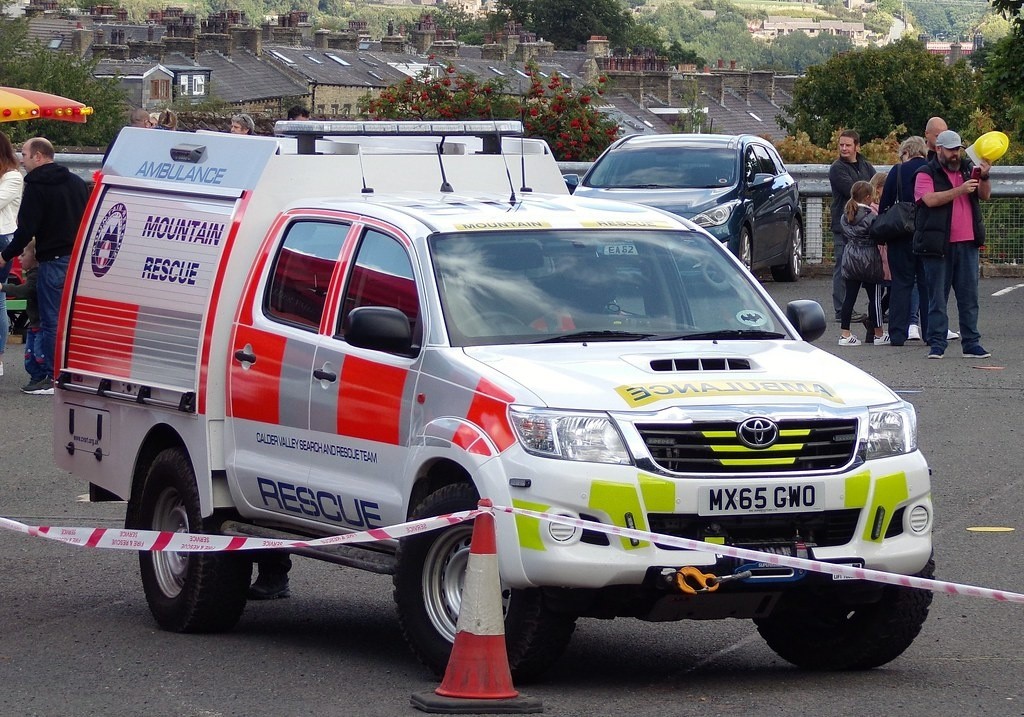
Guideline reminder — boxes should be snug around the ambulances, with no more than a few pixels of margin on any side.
[54,113,940,673]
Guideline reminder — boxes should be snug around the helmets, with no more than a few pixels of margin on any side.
[973,131,1010,163]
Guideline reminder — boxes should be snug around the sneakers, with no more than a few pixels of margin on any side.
[20,380,39,391]
[24,375,55,395]
[908,324,920,339]
[928,348,945,359]
[946,329,960,339]
[838,333,862,346]
[874,332,890,345]
[962,346,991,359]
[834,309,867,323]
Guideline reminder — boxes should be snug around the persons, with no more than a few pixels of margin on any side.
[908,117,959,340]
[129,109,177,130]
[288,106,309,121]
[1,132,90,395]
[839,181,890,346]
[231,114,256,135]
[878,138,929,347]
[863,172,890,343]
[830,130,876,322]
[912,130,992,359]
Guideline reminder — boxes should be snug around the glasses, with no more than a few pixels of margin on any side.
[237,114,247,122]
[144,120,153,126]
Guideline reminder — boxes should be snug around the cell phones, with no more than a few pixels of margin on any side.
[971,166,981,184]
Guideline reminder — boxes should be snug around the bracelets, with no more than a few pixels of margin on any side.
[980,174,989,180]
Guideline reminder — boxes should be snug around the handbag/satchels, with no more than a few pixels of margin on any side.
[869,201,916,243]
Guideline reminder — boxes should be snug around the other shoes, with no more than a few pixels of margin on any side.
[0,362,3,375]
[883,311,890,323]
[865,333,874,343]
[248,570,291,599]
[862,316,869,329]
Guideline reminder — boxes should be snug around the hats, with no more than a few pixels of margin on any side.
[935,130,967,149]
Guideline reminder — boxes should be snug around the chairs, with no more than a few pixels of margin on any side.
[471,242,549,325]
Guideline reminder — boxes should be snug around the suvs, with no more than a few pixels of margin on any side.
[564,132,802,285]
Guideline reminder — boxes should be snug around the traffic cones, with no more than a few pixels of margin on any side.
[410,498,547,715]
